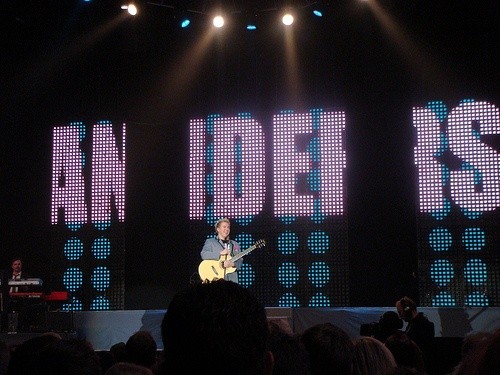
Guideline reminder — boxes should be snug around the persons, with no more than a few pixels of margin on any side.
[1,258,34,334]
[200,217,243,286]
[0,280,500,375]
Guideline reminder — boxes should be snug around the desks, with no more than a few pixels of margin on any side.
[72,305,500,352]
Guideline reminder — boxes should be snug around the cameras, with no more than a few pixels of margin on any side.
[358,311,403,343]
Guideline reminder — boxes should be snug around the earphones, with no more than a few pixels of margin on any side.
[404,306,410,311]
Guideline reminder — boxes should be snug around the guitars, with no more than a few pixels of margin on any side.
[198,239,266,283]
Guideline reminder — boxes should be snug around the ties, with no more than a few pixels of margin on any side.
[13,274,20,279]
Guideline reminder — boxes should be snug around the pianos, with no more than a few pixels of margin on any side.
[0,277,51,332]
